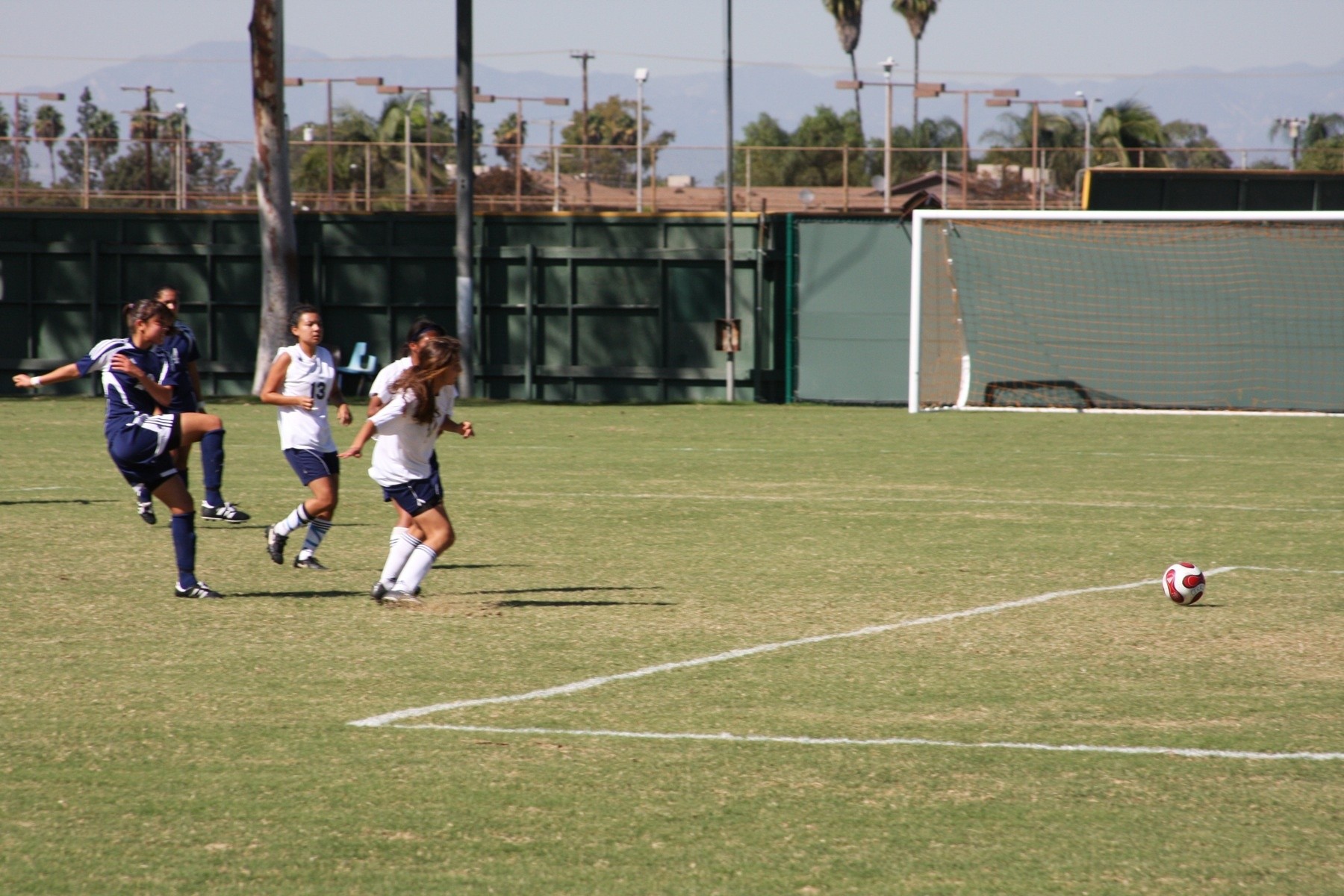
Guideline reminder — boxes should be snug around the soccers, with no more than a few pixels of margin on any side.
[1161,562,1206,606]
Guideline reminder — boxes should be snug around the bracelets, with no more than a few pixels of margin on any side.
[30,376,44,389]
[197,401,206,408]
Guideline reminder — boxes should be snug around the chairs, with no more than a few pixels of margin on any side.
[334,342,378,395]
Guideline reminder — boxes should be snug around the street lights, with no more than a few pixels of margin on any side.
[878,55,897,212]
[471,93,570,212]
[374,84,480,208]
[0,91,66,208]
[284,77,385,195]
[177,104,187,210]
[912,88,1022,210]
[835,79,946,205]
[120,85,175,209]
[1076,90,1105,174]
[633,67,650,212]
[983,96,1088,209]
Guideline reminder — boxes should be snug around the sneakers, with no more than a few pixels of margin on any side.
[294,555,329,571]
[370,578,421,599]
[264,523,290,564]
[381,590,422,606]
[174,580,222,599]
[200,499,250,523]
[134,484,156,525]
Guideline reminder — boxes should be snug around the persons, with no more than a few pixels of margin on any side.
[258,307,354,568]
[368,317,475,593]
[135,287,207,527]
[337,335,476,607]
[11,299,252,598]
